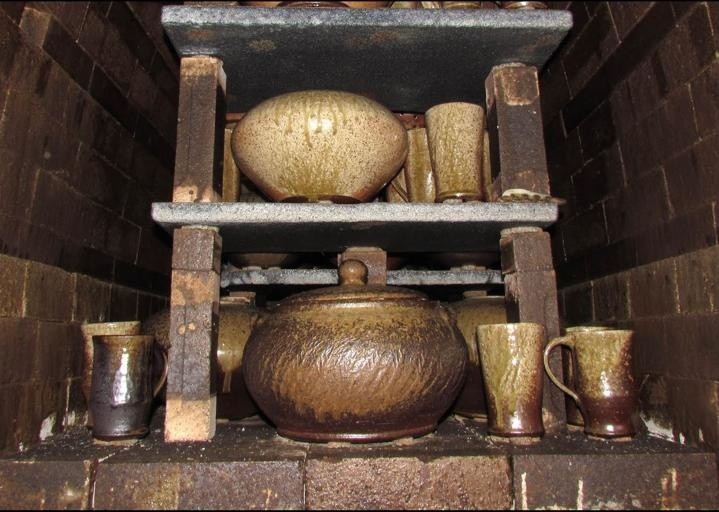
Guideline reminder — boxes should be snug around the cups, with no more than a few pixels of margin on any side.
[387,101,492,202]
[474,322,547,438]
[88,333,169,443]
[77,320,141,421]
[220,126,241,201]
[566,326,613,425]
[543,323,640,438]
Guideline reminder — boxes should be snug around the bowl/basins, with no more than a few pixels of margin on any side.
[229,88,409,203]
[240,298,471,442]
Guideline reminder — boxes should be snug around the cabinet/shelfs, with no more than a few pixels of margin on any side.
[149,1,577,445]
